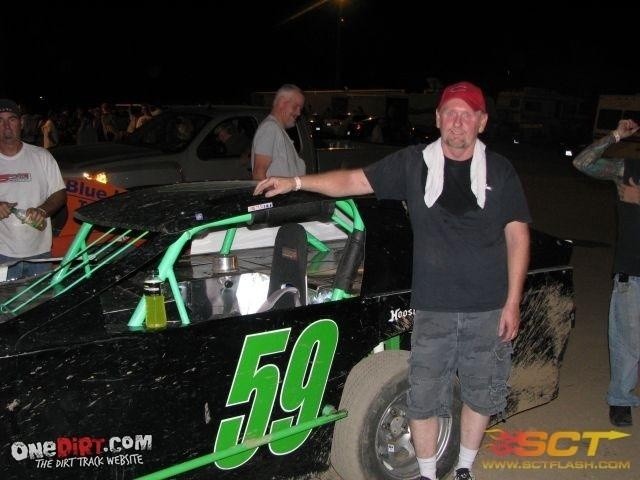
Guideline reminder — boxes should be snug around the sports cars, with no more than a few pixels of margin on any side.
[0,180,575,480]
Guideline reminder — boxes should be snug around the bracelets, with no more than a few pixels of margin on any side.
[36,207,47,215]
[294,176,302,192]
[608,130,620,144]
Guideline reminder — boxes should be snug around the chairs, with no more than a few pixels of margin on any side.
[265,223,310,310]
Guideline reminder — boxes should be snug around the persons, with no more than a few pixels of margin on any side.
[1,101,71,286]
[20,100,254,183]
[250,83,307,182]
[572,116,640,426]
[253,79,532,480]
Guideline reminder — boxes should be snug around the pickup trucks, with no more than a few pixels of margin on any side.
[43,106,400,191]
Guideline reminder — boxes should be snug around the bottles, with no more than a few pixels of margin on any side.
[143,268,167,329]
[9,206,47,231]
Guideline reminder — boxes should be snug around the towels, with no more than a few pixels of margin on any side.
[421,136,488,212]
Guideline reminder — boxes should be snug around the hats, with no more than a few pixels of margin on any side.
[438,82,486,112]
[0,99,21,116]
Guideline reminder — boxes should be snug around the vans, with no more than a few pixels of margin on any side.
[592,94,640,160]
